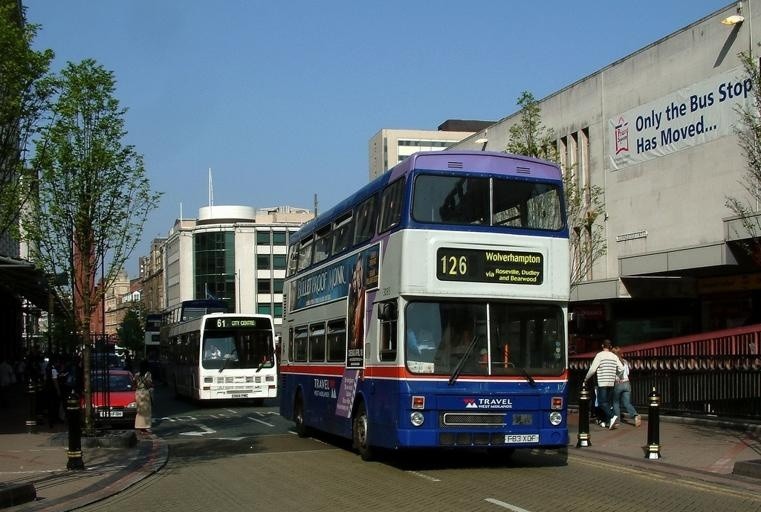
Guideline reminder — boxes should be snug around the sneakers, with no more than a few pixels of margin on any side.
[635,415,642,428]
[600,422,605,428]
[608,415,619,430]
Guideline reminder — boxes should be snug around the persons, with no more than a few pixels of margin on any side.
[133,358,154,434]
[206,345,222,360]
[0,345,80,418]
[584,339,641,430]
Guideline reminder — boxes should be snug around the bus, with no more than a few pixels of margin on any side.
[280,150,571,462]
[144,299,278,407]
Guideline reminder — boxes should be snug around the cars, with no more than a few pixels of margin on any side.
[72,353,139,433]
